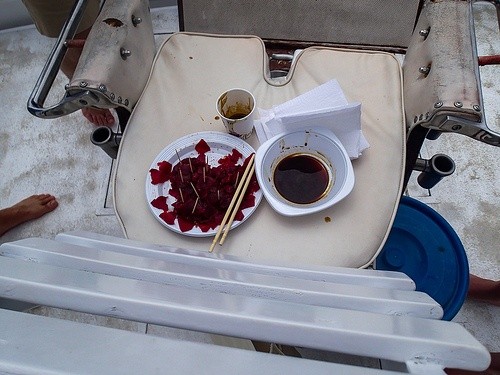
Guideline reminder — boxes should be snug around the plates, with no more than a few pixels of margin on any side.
[145,131,264,238]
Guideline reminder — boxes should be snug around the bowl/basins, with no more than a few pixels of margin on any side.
[253,126,356,217]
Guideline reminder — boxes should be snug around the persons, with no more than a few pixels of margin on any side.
[443,272,500,375]
[23,1,118,126]
[0,193,59,237]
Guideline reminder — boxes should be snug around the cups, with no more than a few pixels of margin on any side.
[216,88,256,140]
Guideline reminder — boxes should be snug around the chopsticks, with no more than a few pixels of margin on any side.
[209,153,255,253]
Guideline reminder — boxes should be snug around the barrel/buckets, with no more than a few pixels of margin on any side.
[375,196,470,323]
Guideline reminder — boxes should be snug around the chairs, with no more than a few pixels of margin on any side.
[27,0,500,269]
